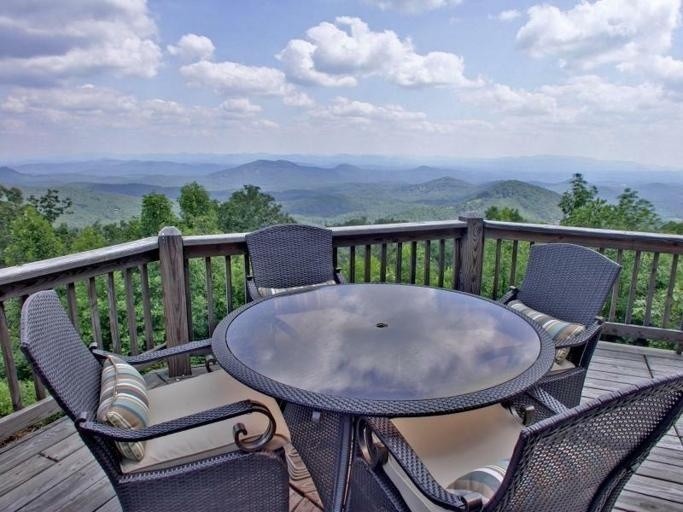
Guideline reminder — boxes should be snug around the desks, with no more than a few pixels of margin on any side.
[211,282,557,511]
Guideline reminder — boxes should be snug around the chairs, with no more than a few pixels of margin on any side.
[20,289,289,512]
[344,374,683,512]
[246,223,346,301]
[496,243,622,426]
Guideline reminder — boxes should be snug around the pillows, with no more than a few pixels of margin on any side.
[508,300,586,365]
[446,446,612,509]
[95,356,150,462]
[260,282,337,296]
